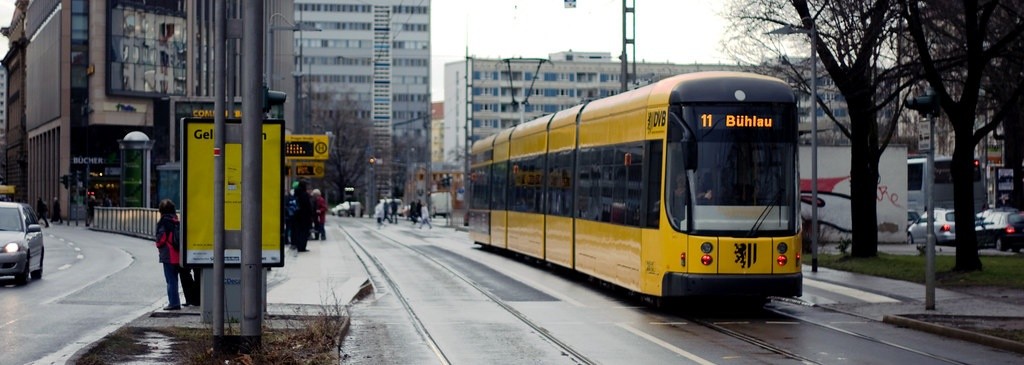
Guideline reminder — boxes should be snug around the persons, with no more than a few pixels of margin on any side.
[36,198,49,228]
[410,198,436,229]
[50,197,63,224]
[154,199,201,310]
[374,197,397,230]
[88,193,117,225]
[285,178,328,252]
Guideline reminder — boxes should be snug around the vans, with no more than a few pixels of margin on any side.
[431,192,452,218]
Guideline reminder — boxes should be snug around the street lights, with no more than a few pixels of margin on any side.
[763,24,818,273]
[268,23,322,89]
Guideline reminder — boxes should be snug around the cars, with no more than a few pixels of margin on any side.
[380,198,404,217]
[332,202,363,217]
[907,206,981,249]
[0,203,44,289]
[975,208,1023,251]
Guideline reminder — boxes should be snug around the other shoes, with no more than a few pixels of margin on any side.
[181,301,197,306]
[163,305,180,310]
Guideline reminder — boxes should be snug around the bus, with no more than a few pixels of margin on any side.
[907,157,989,225]
[467,72,802,312]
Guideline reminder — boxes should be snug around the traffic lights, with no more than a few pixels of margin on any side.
[77,163,84,184]
[60,174,69,189]
[905,93,940,119]
[263,85,287,115]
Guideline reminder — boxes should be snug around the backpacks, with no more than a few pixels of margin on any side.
[159,217,180,252]
